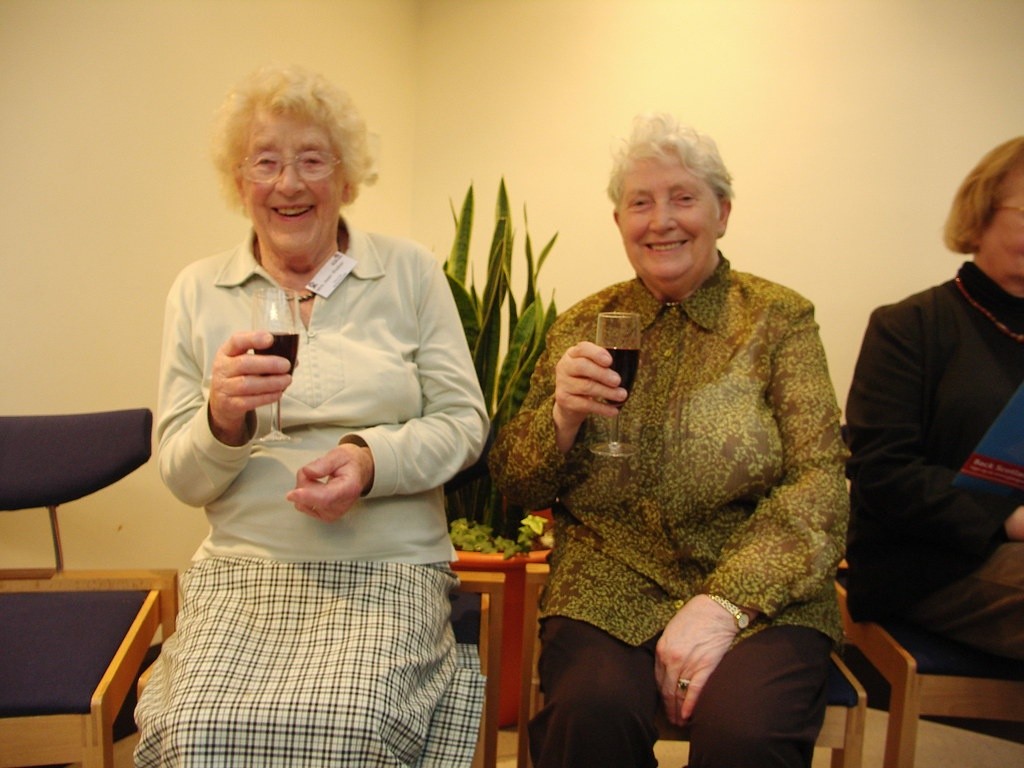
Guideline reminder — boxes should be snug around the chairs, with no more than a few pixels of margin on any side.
[450,568,1023,767]
[1,409,178,768]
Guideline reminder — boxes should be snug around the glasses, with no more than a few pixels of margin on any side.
[239,149,342,185]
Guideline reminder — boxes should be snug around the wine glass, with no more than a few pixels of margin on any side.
[590,310,642,457]
[249,287,302,448]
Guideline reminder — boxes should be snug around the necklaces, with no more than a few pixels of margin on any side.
[286,293,314,301]
[957,277,1024,341]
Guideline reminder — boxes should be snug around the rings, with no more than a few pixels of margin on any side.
[312,507,315,511]
[678,680,688,688]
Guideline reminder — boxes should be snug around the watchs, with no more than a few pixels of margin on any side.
[709,594,750,630]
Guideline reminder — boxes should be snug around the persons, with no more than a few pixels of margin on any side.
[487,116,849,767]
[844,137,1024,743]
[132,75,490,768]
